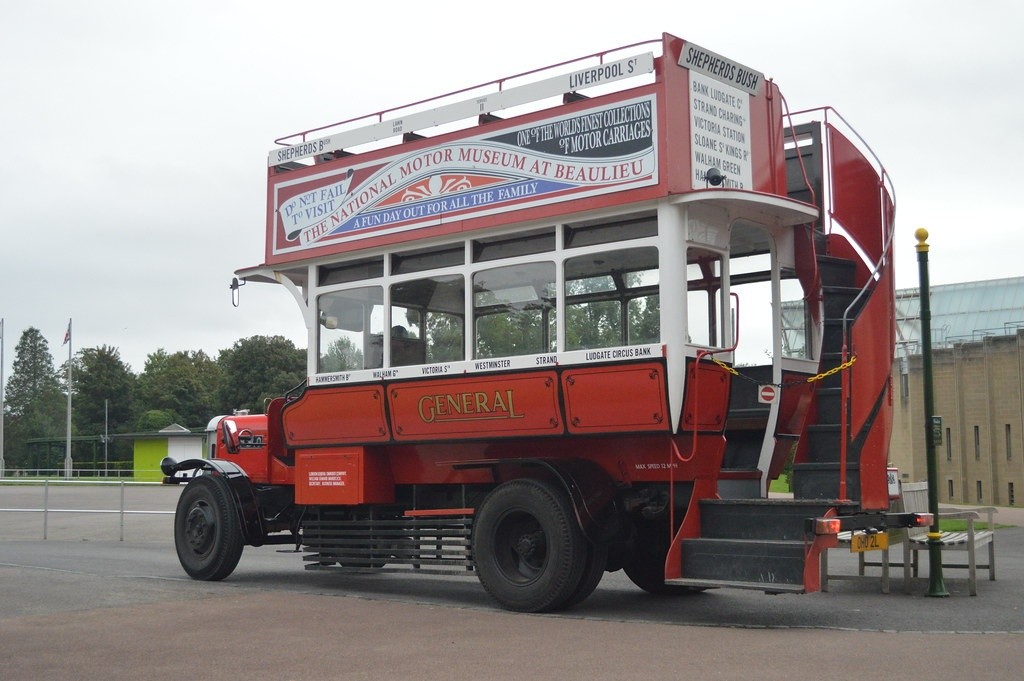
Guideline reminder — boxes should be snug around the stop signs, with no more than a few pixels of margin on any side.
[759,384,778,403]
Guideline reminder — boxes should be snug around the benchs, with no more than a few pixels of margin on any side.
[820,498,919,594]
[897,479,997,596]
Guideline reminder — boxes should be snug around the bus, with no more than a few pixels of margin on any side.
[160,30,934,614]
